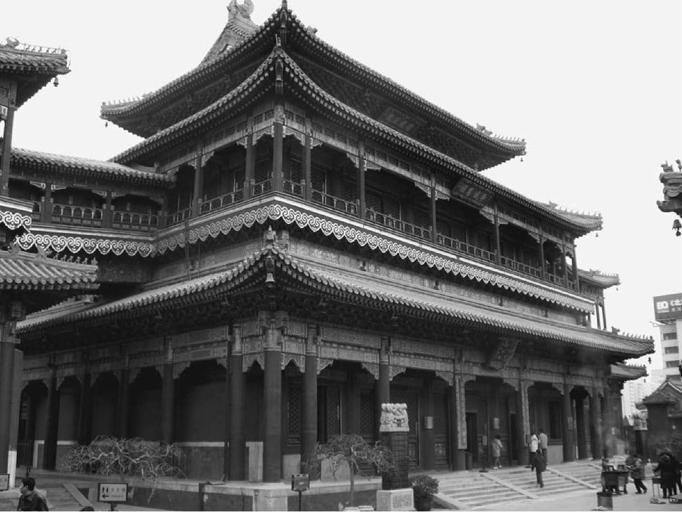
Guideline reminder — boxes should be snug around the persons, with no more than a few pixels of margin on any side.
[17,478,48,511]
[490,434,503,469]
[526,427,548,488]
[629,453,682,498]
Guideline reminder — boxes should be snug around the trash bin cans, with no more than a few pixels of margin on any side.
[597,492,612,511]
